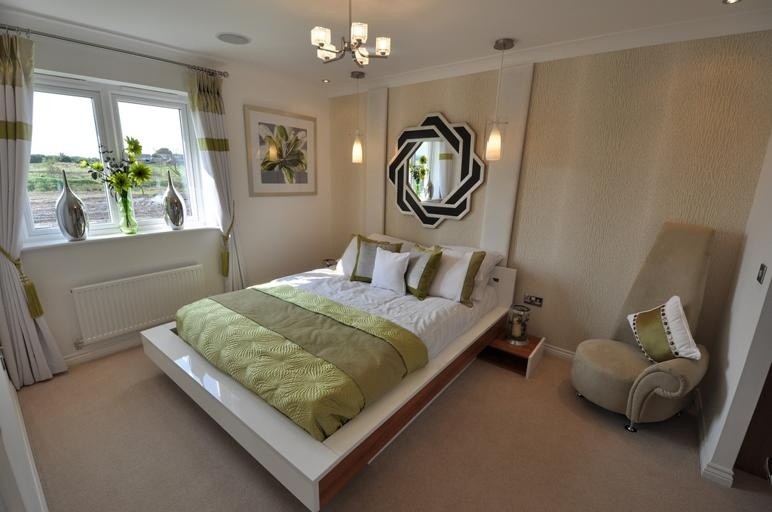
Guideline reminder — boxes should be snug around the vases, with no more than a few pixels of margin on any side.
[115,197,139,233]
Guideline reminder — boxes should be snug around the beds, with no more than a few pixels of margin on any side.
[140,232,517,511]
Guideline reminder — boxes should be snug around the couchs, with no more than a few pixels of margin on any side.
[570,223,714,434]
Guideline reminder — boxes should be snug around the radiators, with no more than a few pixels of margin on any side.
[70,264,204,349]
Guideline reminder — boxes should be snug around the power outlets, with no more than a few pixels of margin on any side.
[523,293,545,308]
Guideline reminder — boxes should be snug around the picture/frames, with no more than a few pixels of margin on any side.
[243,102,319,197]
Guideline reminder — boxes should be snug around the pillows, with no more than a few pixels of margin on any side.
[371,248,413,295]
[335,234,504,304]
[401,241,444,298]
[430,249,486,304]
[351,234,400,283]
[624,295,703,362]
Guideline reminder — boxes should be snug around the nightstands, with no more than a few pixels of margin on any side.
[491,329,549,379]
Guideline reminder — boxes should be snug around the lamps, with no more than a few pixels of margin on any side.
[348,72,368,166]
[310,3,391,68]
[486,35,513,163]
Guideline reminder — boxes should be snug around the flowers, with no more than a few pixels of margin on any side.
[410,154,429,199]
[76,135,153,193]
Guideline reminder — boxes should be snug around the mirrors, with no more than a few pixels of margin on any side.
[381,112,485,230]
